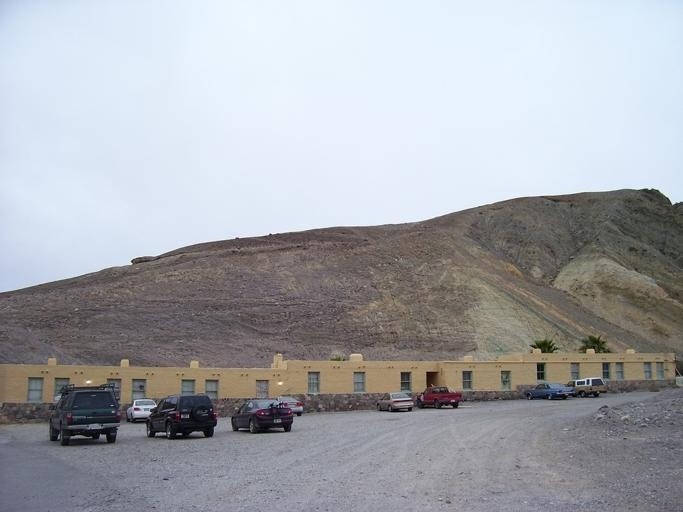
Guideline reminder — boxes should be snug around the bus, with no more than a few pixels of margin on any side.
[377,392,414,412]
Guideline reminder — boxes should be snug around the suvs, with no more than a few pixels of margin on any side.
[146,394,217,440]
[49,383,122,445]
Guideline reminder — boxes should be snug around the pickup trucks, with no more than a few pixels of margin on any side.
[416,386,462,409]
[567,377,608,398]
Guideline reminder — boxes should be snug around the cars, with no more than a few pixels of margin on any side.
[126,399,157,423]
[275,397,304,416]
[524,383,576,400]
[231,400,293,434]
